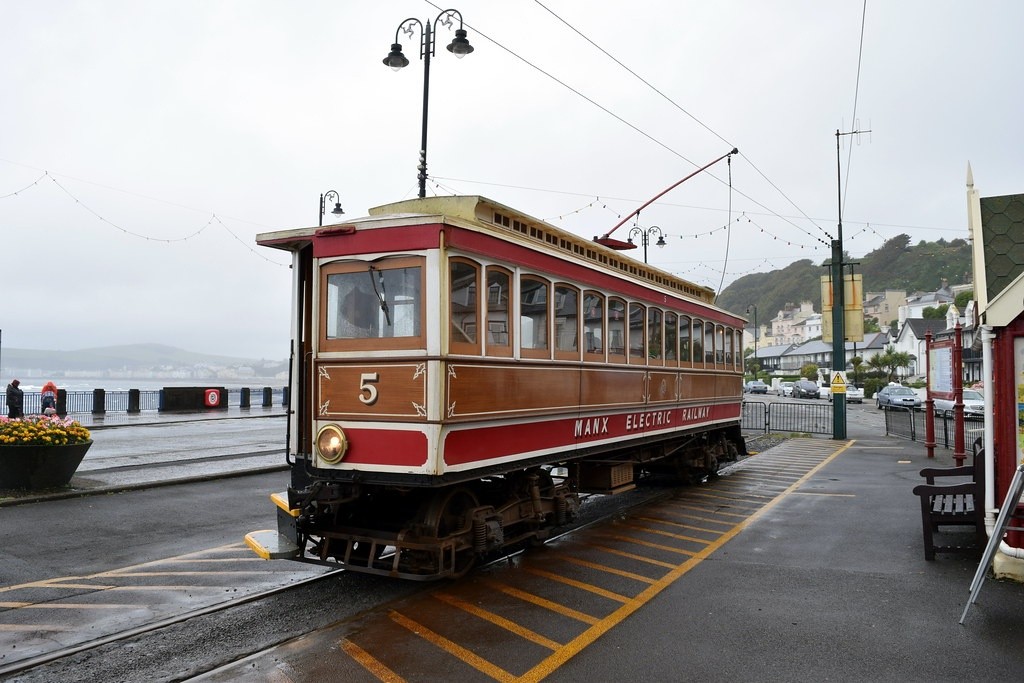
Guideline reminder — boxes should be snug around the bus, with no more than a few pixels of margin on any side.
[257,196,752,582]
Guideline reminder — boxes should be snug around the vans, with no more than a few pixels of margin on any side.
[934,389,986,420]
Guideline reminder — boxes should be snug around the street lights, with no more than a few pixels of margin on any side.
[627,226,667,265]
[382,8,474,199]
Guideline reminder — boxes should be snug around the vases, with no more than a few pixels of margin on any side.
[0,438,94,491]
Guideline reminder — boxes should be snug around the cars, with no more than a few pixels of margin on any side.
[776,382,795,397]
[791,380,821,399]
[876,386,921,411]
[746,381,767,394]
[828,385,863,403]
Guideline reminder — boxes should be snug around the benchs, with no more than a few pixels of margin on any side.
[914,437,987,562]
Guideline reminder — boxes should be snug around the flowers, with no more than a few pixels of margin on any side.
[0,413,92,445]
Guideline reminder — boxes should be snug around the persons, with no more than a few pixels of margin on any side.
[41,382,57,413]
[6,380,25,418]
